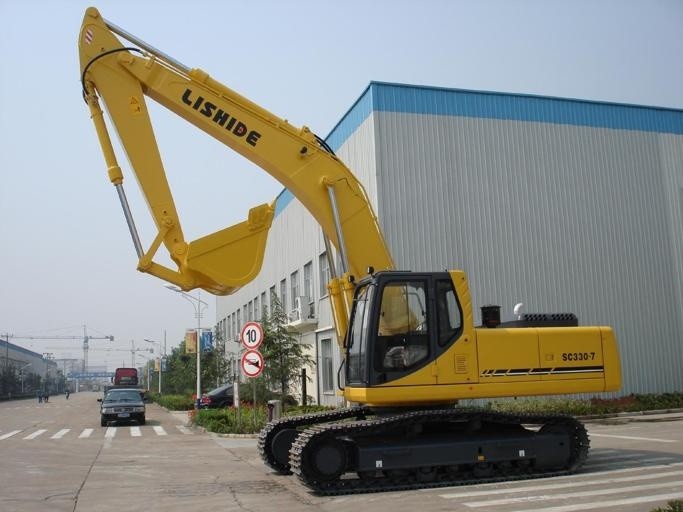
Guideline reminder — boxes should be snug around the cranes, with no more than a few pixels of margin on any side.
[8,325,113,382]
[114,336,151,366]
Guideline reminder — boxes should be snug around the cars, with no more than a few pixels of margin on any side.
[98,386,146,427]
[194,382,297,411]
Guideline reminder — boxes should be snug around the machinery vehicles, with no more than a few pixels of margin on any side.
[78,6,621,497]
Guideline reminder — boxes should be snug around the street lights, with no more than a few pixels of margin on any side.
[165,284,201,412]
[143,337,161,397]
[18,363,31,392]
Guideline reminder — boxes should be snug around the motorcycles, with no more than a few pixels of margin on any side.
[64,392,69,399]
[39,395,49,404]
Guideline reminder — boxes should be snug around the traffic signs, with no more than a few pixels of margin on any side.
[240,322,263,350]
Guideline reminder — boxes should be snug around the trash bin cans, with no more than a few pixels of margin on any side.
[268,400,282,422]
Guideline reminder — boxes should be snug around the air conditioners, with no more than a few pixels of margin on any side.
[294,295,310,319]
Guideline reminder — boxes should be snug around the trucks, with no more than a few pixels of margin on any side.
[107,367,139,389]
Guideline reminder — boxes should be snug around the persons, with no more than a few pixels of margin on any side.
[65,389,70,400]
[36,391,49,403]
